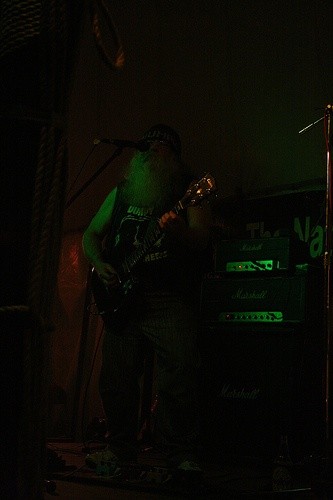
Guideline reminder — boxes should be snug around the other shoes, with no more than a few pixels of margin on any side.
[84,445,127,474]
[167,454,203,473]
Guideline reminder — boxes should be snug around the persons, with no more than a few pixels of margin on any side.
[83,125,197,482]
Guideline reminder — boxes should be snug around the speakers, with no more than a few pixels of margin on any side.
[94,138,129,147]
[192,324,327,458]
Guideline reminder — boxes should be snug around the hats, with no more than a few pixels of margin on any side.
[139,126,182,157]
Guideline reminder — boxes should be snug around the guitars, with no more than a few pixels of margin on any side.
[90,171,218,334]
